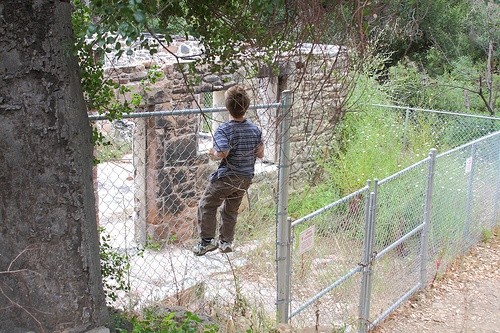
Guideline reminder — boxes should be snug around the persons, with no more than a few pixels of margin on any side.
[192,85,264,256]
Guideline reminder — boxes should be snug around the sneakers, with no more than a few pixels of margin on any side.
[192,238,219,256]
[217,239,233,253]
